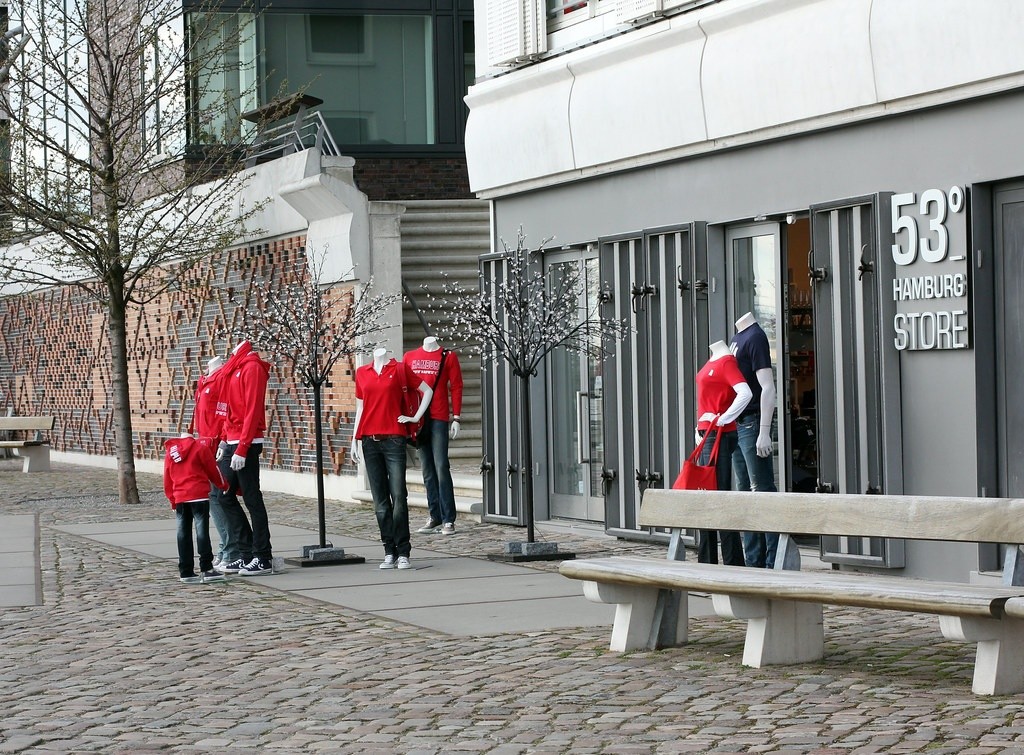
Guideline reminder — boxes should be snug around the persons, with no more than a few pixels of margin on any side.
[403,337,463,535]
[730,312,780,569]
[195,356,237,571]
[351,349,433,570]
[695,340,752,568]
[221,341,273,576]
[163,434,230,582]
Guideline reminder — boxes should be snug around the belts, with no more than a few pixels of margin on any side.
[367,433,399,441]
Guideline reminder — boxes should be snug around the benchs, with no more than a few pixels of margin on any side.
[558,488,1024,698]
[0,415,57,474]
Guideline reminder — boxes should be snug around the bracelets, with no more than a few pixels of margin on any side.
[453,418,460,424]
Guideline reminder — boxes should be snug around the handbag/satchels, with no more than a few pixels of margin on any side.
[193,437,218,457]
[405,408,431,449]
[400,363,425,441]
[672,417,723,490]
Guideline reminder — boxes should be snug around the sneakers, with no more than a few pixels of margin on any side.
[419,518,442,533]
[398,556,411,569]
[442,522,455,534]
[204,570,224,581]
[220,559,245,573]
[181,574,199,581]
[238,557,272,575]
[379,555,397,568]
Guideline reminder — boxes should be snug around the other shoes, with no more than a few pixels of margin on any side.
[216,561,231,567]
[213,558,221,565]
